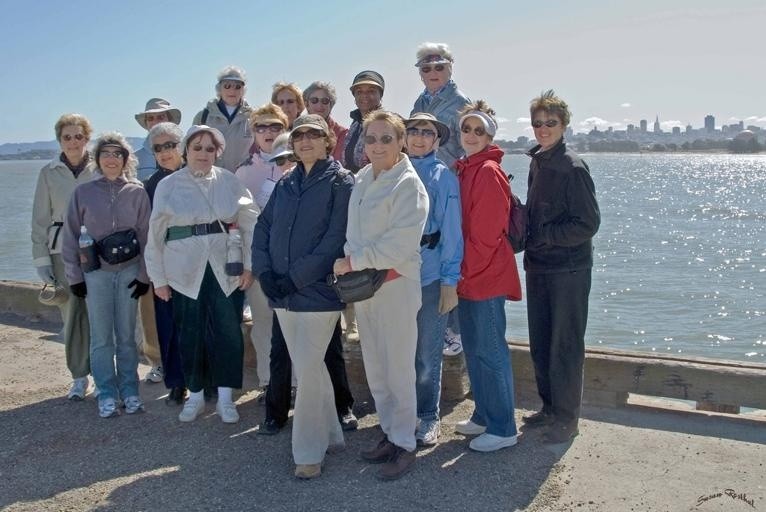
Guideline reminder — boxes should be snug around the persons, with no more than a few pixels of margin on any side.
[521,87,602,444]
[60,130,151,418]
[241,82,305,321]
[235,103,297,408]
[334,110,430,480]
[142,122,212,405]
[30,115,106,401]
[409,45,472,359]
[257,130,359,434]
[404,112,465,445]
[340,70,384,344]
[131,98,182,383]
[145,129,261,422]
[302,81,349,168]
[192,65,255,176]
[251,114,355,478]
[449,101,521,451]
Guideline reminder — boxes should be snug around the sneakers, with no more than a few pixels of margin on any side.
[454,419,487,435]
[365,418,439,479]
[97,396,145,418]
[442,327,463,355]
[544,421,579,442]
[522,410,554,426]
[468,434,517,451]
[69,376,89,400]
[145,366,163,385]
[163,388,240,425]
[256,385,357,478]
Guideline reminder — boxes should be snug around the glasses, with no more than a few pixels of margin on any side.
[291,128,321,142]
[146,113,165,121]
[224,83,241,90]
[365,135,393,144]
[422,65,444,73]
[406,126,435,136]
[462,125,484,135]
[256,123,283,133]
[63,134,214,157]
[274,97,330,105]
[531,119,558,128]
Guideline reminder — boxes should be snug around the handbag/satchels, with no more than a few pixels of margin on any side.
[97,228,141,266]
[328,268,390,304]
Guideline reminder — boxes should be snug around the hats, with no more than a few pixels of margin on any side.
[220,73,245,84]
[252,113,287,128]
[289,114,329,142]
[135,98,181,134]
[459,110,498,137]
[415,54,451,67]
[182,124,226,159]
[350,71,384,91]
[405,112,450,146]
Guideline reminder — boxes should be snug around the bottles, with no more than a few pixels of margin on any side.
[225,223,244,276]
[78,225,101,273]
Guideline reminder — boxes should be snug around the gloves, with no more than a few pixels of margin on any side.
[70,281,87,300]
[127,279,150,301]
[260,270,296,303]
[36,265,56,283]
[438,286,459,314]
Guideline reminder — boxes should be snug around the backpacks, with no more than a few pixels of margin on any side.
[508,193,529,254]
